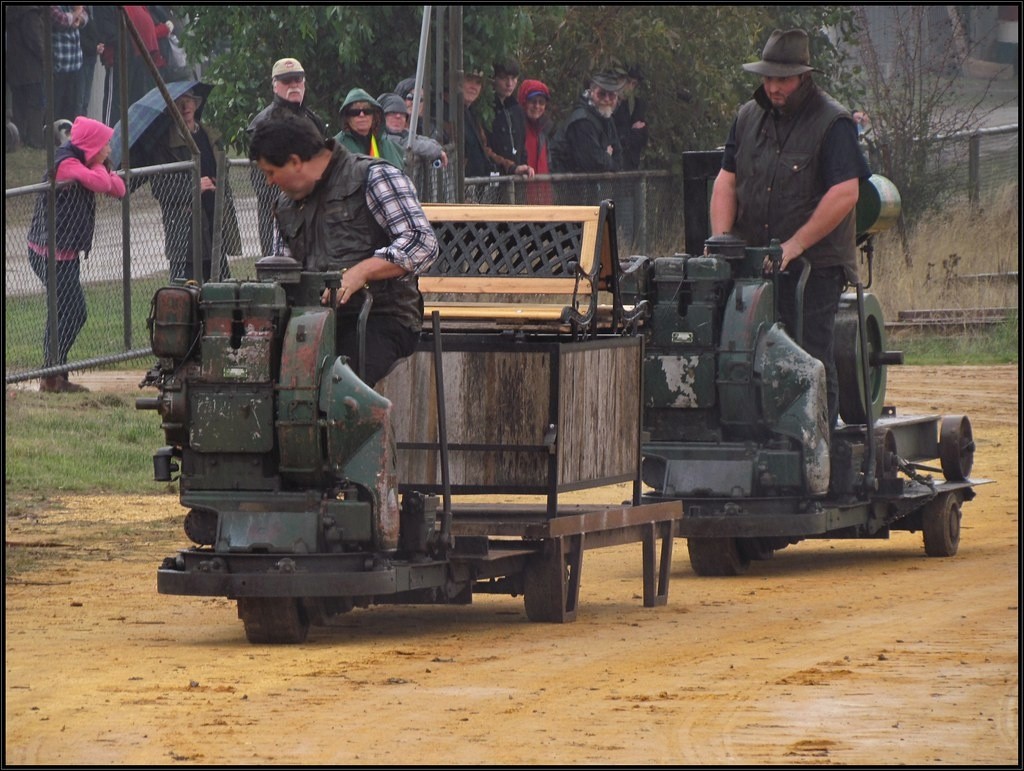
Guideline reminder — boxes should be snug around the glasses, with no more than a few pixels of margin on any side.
[595,91,617,100]
[346,107,375,117]
[275,76,303,84]
[407,93,425,102]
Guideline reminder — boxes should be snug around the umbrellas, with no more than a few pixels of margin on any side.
[105,80,214,196]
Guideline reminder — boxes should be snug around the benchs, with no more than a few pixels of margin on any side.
[418,199,652,343]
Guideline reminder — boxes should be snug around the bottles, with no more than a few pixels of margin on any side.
[433,159,442,169]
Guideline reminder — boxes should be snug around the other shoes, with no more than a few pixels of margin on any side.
[38,374,90,393]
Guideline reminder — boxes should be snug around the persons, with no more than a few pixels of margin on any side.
[28,117,125,391]
[5,5,649,285]
[249,115,439,389]
[703,28,873,442]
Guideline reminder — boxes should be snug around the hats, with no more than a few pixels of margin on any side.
[271,58,305,81]
[590,68,627,92]
[526,91,547,100]
[164,88,203,115]
[741,28,827,77]
